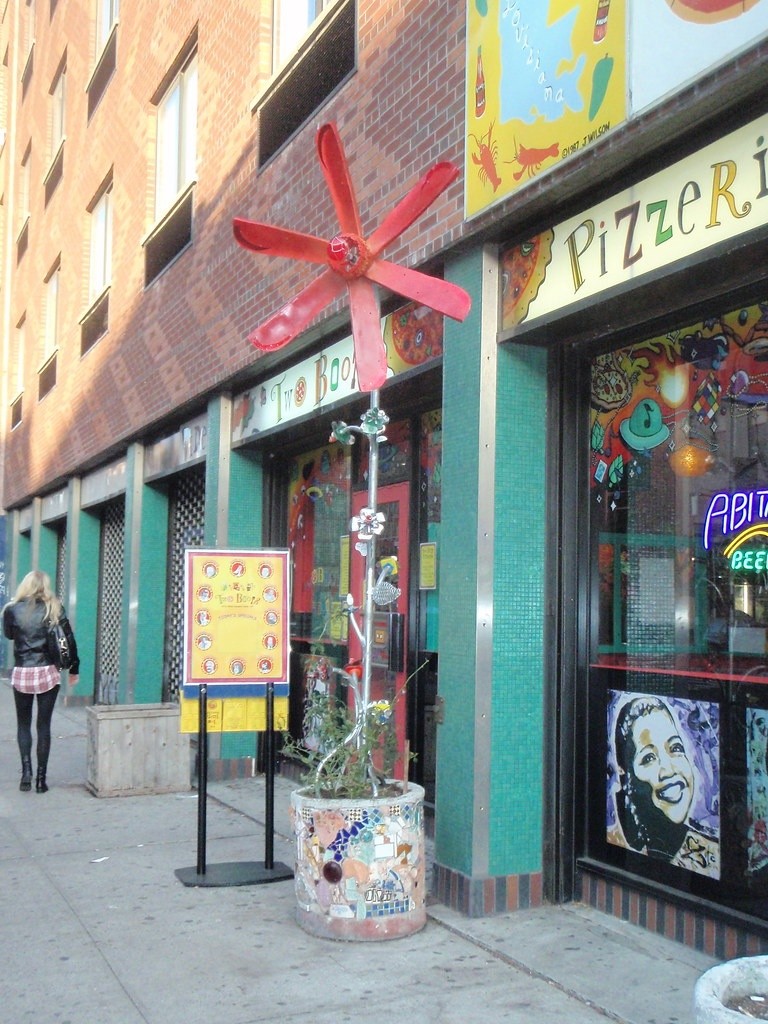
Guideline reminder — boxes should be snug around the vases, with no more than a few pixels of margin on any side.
[291,778,427,941]
[84,703,192,799]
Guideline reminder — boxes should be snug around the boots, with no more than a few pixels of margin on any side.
[20,760,32,791]
[37,766,48,792]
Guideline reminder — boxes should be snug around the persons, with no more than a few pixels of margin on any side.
[0,570,80,793]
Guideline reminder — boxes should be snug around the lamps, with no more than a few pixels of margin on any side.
[663,420,720,478]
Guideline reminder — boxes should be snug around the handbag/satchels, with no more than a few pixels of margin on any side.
[47,624,71,671]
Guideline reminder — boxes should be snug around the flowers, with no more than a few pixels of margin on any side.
[275,610,419,798]
